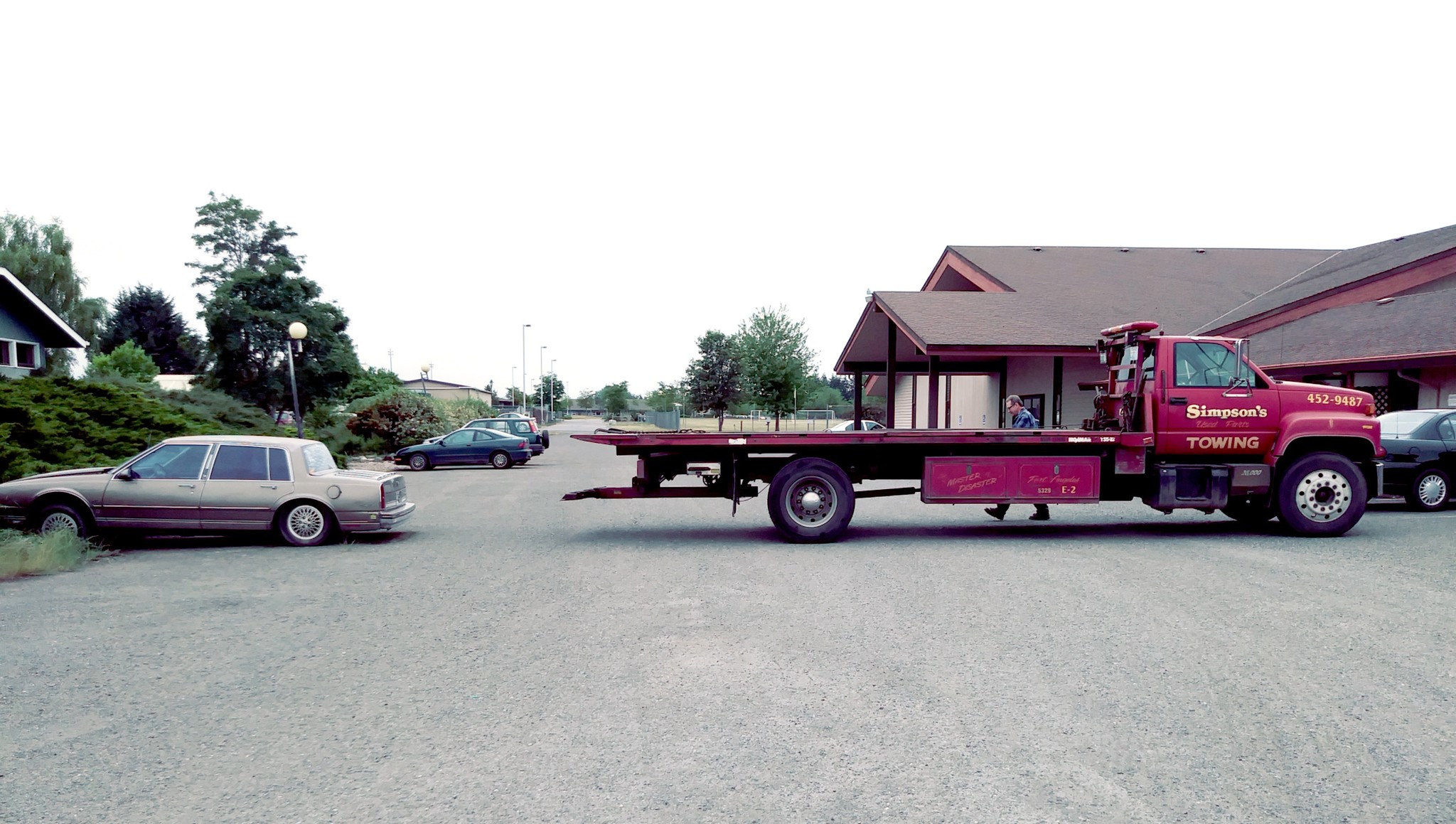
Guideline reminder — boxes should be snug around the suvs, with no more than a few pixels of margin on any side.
[423,417,550,466]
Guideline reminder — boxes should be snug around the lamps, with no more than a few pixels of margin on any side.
[866,288,873,302]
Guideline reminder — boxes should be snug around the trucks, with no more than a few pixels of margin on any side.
[559,321,1384,542]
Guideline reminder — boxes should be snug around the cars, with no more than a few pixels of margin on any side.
[1375,407,1456,512]
[492,413,537,431]
[274,411,293,425]
[0,435,416,548]
[826,420,886,432]
[760,415,765,419]
[747,415,754,418]
[393,427,532,471]
[723,413,728,416]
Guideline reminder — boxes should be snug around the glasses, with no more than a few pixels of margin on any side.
[1008,404,1015,410]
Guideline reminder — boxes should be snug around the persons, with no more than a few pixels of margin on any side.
[984,394,1050,520]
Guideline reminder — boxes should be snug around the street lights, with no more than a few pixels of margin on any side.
[551,360,557,420]
[490,379,494,391]
[540,346,547,424]
[286,322,307,438]
[421,364,430,394]
[512,367,517,406]
[523,324,531,413]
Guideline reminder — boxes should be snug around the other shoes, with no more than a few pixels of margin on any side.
[1029,509,1049,520]
[984,508,1006,520]
